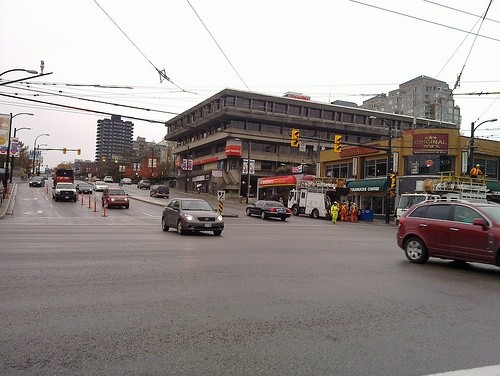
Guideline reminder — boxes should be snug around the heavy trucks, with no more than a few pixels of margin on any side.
[52,169,74,187]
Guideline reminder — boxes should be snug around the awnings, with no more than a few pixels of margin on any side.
[259,176,297,187]
[348,179,391,192]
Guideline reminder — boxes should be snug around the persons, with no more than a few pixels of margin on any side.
[279,196,288,207]
[470,164,483,184]
[331,201,339,224]
[338,200,358,222]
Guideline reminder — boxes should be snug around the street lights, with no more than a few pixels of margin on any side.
[467,118,497,174]
[9,127,31,183]
[5,112,34,180]
[235,139,250,204]
[369,115,391,224]
[177,142,189,193]
[34,134,50,148]
[39,144,48,147]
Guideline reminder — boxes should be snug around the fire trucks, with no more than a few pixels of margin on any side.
[288,177,350,219]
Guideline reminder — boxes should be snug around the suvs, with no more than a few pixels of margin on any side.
[101,187,129,209]
[150,186,170,199]
[137,180,153,190]
[52,182,79,202]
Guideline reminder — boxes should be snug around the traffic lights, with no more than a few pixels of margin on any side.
[390,174,396,198]
[78,150,80,155]
[291,129,300,147]
[334,135,342,152]
[63,148,66,154]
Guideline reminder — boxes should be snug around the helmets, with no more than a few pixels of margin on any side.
[334,201,337,204]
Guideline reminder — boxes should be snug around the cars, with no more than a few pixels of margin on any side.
[104,176,113,183]
[76,183,92,194]
[86,177,89,181]
[161,198,224,236]
[95,178,100,180]
[29,176,45,187]
[123,178,132,185]
[93,181,108,192]
[245,200,292,221]
[396,198,500,268]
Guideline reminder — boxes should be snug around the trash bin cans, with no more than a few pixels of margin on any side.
[358,209,374,222]
